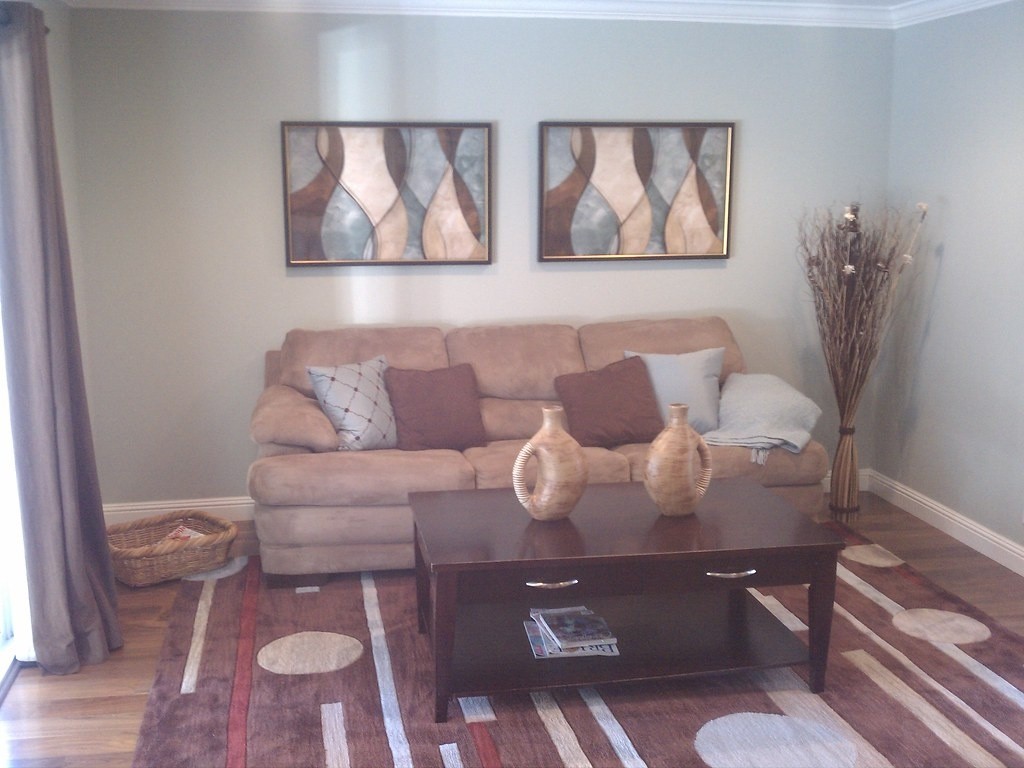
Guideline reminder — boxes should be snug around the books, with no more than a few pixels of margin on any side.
[523,606,620,659]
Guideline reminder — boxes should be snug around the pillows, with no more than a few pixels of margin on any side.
[382,361,487,450]
[305,355,398,452]
[620,344,724,435]
[554,357,666,450]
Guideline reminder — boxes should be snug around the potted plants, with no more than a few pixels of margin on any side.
[794,202,928,523]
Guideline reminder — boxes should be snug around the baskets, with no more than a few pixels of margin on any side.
[104,510,238,587]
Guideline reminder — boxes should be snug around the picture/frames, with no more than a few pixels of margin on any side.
[537,121,734,262]
[281,120,493,267]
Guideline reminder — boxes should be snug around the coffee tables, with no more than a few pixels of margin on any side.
[406,478,846,726]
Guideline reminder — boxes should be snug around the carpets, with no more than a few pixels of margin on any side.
[128,523,1024,768]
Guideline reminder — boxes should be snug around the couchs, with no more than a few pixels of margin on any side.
[247,317,829,589]
